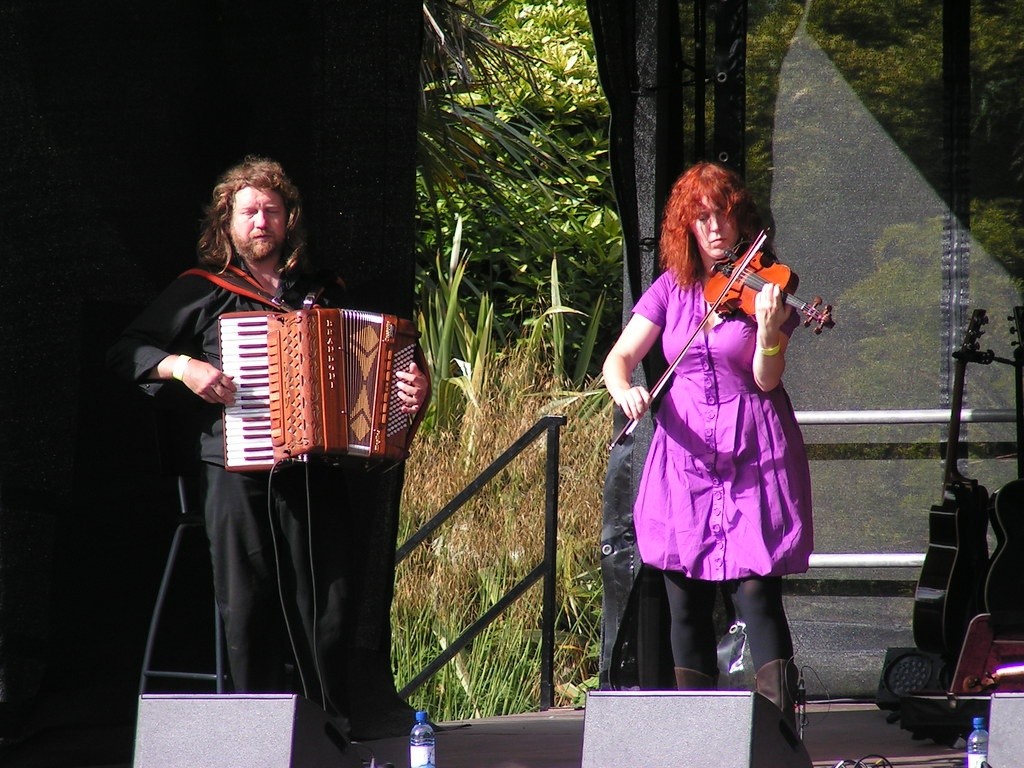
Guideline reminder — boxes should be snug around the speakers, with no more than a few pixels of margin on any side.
[133,696,370,768]
[580,687,815,767]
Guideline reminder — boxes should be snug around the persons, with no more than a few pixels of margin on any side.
[110,155,431,744]
[601,162,815,713]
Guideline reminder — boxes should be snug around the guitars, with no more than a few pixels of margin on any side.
[911,307,995,656]
[974,306,1024,633]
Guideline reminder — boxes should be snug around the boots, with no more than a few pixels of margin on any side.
[673,659,797,735]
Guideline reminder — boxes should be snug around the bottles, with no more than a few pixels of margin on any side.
[967,717,988,768]
[409,712,437,768]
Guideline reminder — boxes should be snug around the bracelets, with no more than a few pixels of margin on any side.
[173,354,192,381]
[755,339,781,356]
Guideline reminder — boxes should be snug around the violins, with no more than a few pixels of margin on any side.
[703,237,836,336]
